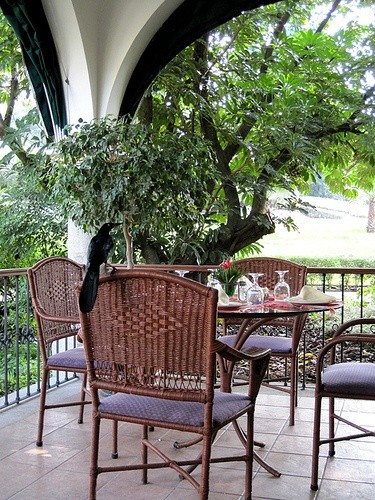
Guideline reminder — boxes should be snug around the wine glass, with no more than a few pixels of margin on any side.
[175,268,190,278]
[205,268,221,289]
[273,270,290,300]
[245,272,266,306]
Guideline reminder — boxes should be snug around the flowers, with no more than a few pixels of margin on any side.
[212,258,245,282]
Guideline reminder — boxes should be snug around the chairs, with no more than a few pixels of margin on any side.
[207,255,308,426]
[26,256,153,458]
[74,270,272,500]
[310,317,375,490]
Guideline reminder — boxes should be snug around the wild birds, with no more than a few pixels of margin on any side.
[78,222,123,315]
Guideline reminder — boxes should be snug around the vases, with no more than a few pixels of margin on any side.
[224,284,236,296]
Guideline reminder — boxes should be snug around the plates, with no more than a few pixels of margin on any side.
[218,301,243,310]
[287,295,337,307]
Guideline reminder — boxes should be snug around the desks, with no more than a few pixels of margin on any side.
[173,294,344,480]
